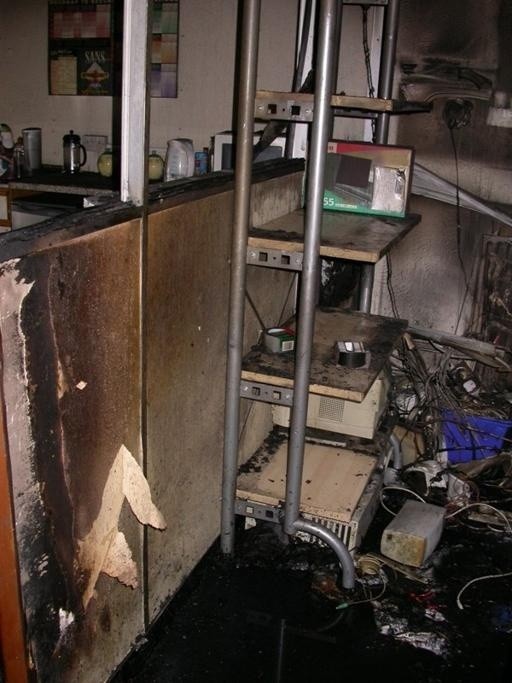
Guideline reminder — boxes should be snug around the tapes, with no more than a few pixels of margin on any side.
[263,326,296,355]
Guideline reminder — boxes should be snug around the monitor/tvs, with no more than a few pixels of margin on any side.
[210,130,285,175]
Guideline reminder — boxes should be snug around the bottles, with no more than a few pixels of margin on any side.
[62,130,87,173]
[22,128,41,169]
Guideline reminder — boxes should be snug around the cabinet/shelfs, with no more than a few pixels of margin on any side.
[219,1,433,586]
